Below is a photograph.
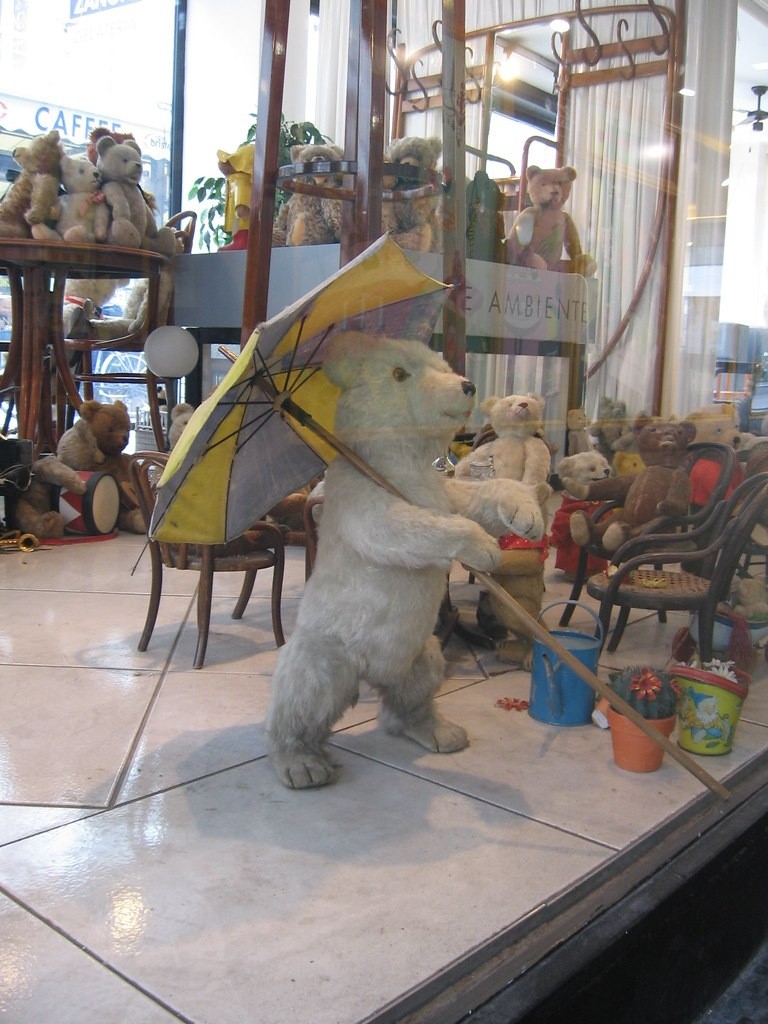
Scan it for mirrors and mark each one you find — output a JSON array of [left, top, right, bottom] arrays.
[[482, 17, 570, 181]]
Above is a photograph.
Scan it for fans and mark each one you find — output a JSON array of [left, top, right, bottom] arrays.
[[731, 86, 768, 133]]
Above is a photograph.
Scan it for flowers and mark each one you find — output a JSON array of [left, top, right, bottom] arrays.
[[606, 668, 679, 718], [679, 657, 739, 685]]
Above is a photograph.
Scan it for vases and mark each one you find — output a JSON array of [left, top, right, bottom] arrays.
[[671, 666, 752, 754], [605, 704, 677, 773]]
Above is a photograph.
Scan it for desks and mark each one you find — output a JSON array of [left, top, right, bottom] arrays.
[[0, 236, 167, 462]]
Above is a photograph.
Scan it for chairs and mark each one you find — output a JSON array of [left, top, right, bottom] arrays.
[[127, 449, 327, 671], [559, 441, 768, 666], [0, 211, 198, 452]]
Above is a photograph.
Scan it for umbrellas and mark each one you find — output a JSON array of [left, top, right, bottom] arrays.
[[147, 233, 735, 806]]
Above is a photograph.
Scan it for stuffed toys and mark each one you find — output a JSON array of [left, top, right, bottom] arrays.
[[270, 332, 543, 790], [0, 129, 767, 640]]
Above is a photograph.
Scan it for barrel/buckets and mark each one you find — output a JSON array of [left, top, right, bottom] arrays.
[[674, 661, 748, 756]]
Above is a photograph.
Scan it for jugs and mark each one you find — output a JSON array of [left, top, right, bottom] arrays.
[[525, 599, 605, 727]]
